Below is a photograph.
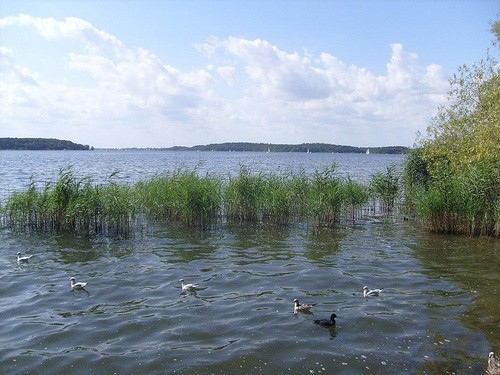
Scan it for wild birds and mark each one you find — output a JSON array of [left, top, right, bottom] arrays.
[[313, 313, 339, 327], [17, 251, 29, 263], [363, 285, 384, 298], [179, 279, 199, 295], [293, 298, 318, 313], [69, 276, 87, 291], [487, 351, 500, 375]]
